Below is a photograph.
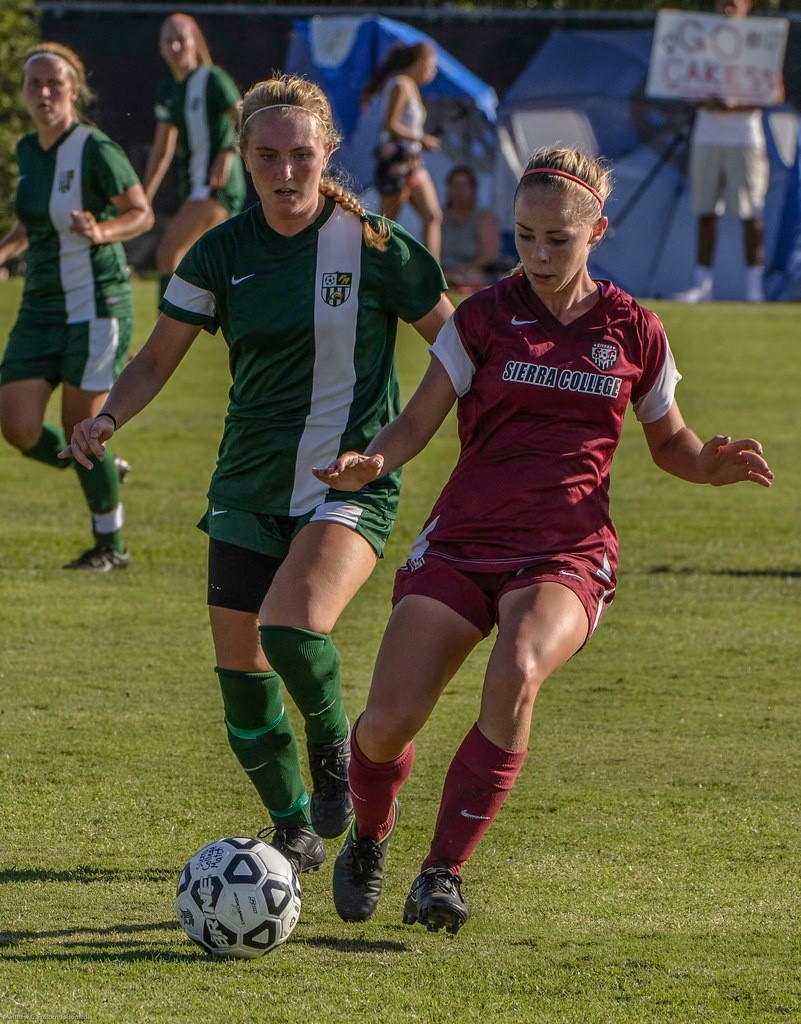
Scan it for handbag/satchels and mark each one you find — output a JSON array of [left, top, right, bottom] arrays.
[[372, 137, 421, 196]]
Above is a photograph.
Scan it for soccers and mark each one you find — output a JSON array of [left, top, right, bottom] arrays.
[[176, 837, 301, 961]]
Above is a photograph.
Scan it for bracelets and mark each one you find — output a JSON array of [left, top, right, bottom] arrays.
[[94, 412, 118, 431]]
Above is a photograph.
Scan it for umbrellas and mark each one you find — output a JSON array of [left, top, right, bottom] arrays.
[[283, 14, 527, 290], [495, 32, 800, 301]]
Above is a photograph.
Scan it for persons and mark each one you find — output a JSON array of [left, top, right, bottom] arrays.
[[359, 37, 444, 270], [57, 79, 458, 873], [141, 14, 246, 316], [357, 130, 500, 297], [311, 149, 775, 937], [672, 0, 786, 306], [0, 42, 155, 575]]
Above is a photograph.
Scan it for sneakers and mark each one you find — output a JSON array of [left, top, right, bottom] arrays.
[[745, 279, 765, 303], [673, 276, 712, 304], [333, 795, 399, 922], [309, 717, 354, 836], [270, 824, 326, 873], [113, 456, 130, 484], [401, 869, 470, 936], [61, 546, 129, 572]]
